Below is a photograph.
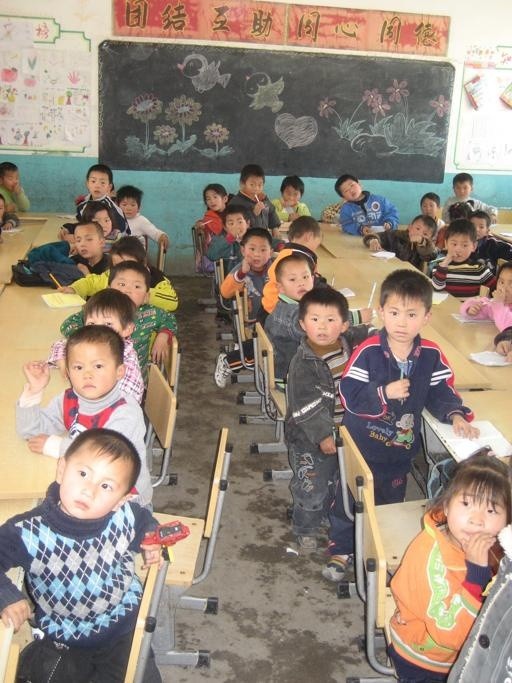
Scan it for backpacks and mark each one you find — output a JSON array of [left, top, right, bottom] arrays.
[[194, 219, 215, 273], [11, 258, 51, 288]]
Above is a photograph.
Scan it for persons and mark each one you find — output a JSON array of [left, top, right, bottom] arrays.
[[0, 426, 166, 683], [282, 287, 383, 553], [13, 325, 157, 513], [444, 519, 512, 682], [386, 444, 512, 683], [322, 267, 482, 582]]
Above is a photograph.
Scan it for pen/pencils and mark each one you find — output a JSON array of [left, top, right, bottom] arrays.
[[47, 271, 62, 288], [368, 281, 377, 308], [401, 368, 404, 405], [332, 272, 335, 286], [255, 194, 260, 202], [202, 220, 212, 225]]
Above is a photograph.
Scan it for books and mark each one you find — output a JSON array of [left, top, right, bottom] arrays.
[[434, 415, 510, 463]]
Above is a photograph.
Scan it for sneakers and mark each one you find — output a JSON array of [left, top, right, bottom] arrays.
[[214, 353, 232, 389], [297, 534, 317, 555], [214, 314, 235, 332], [322, 552, 354, 582]]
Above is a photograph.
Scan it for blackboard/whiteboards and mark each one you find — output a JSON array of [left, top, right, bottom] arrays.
[[99, 41, 456, 185]]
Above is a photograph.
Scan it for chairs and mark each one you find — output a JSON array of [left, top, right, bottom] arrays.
[[125, 427, 233, 683], [156, 239, 168, 273], [192, 221, 225, 314], [214, 257, 257, 384], [137, 335, 181, 488], [333, 425, 435, 678], [236, 321, 295, 481]]
